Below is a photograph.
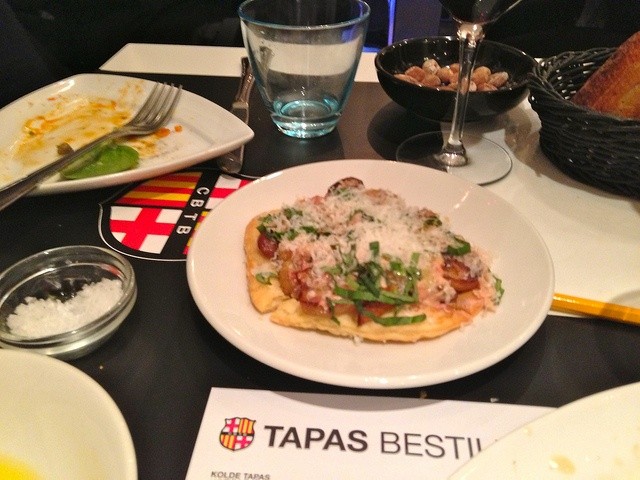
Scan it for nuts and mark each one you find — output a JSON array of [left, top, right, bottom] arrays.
[[394, 57, 510, 94]]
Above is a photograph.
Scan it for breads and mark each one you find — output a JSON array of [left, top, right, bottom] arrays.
[[570, 30, 639, 121]]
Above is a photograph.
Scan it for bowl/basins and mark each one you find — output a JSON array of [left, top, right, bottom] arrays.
[[374, 35, 538, 124], [0, 244, 136, 360]]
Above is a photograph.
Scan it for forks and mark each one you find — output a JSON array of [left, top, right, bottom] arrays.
[[0, 82, 183, 210]]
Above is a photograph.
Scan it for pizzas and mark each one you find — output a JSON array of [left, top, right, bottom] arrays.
[[243, 177, 506, 344]]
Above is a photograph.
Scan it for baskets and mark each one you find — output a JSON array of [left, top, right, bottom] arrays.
[[526, 46, 639, 200]]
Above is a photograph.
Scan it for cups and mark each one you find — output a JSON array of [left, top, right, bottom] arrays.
[[395, 0, 519, 187], [238, 1, 372, 140]]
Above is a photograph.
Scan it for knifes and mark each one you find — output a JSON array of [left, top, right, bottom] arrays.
[[220, 65, 251, 176]]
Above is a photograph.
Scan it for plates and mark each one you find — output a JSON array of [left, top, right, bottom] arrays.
[[447, 381, 640, 480], [0, 349, 138, 480], [2, 73, 257, 197], [185, 158, 555, 390]]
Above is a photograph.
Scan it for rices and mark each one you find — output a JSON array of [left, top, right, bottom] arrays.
[[6, 275, 124, 339]]
